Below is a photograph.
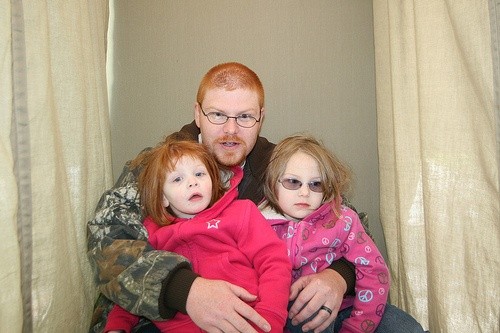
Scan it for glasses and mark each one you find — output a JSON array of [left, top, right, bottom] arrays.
[[198, 101, 262, 128], [277, 179, 326, 193]]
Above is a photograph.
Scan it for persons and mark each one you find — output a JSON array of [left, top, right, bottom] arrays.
[[100, 139, 291, 333], [82, 62, 427, 333], [254, 134, 393, 333]]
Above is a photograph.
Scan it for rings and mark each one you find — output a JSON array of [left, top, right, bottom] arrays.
[[320, 306, 333, 314]]
